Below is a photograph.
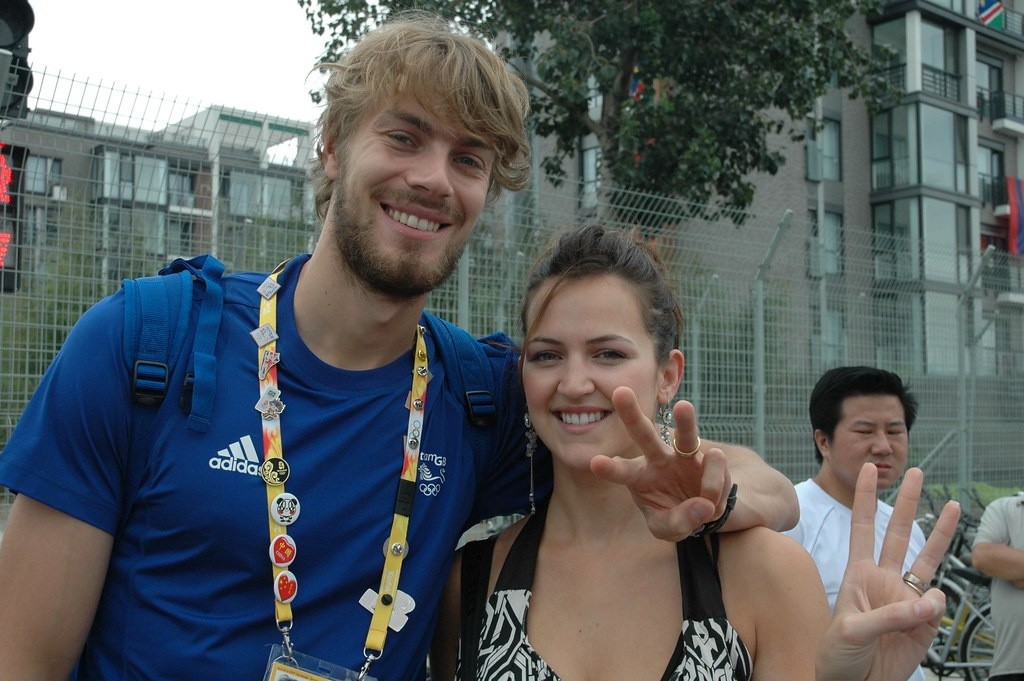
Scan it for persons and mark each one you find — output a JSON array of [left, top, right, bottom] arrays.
[[779, 366, 926, 681], [0, 22, 800, 681], [427, 223, 961, 681], [972, 492, 1024, 681]]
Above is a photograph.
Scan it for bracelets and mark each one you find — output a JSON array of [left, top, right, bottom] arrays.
[[690, 483, 738, 536]]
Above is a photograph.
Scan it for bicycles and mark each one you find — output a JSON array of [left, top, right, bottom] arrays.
[[894, 482, 1024, 681]]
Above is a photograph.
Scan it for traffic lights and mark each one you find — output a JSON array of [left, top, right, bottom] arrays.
[[1, 0, 35, 119], [0, 139, 31, 294]]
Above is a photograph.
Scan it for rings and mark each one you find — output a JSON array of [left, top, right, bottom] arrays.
[[903, 571, 931, 595], [672, 437, 701, 456]]
